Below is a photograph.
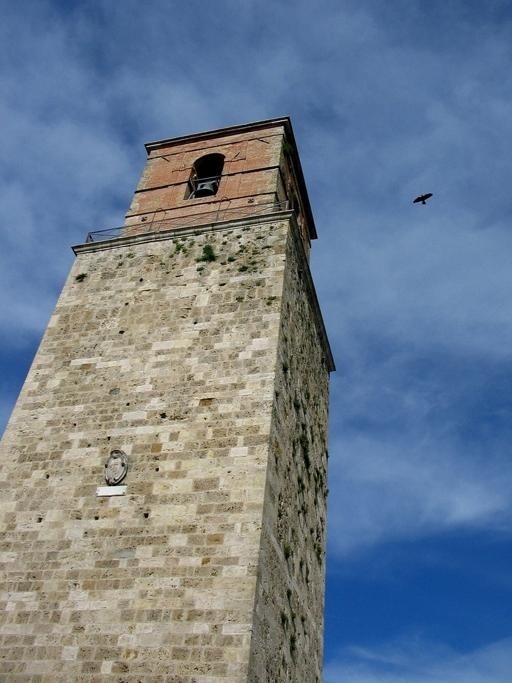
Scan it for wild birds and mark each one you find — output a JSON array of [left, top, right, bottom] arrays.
[[413, 193, 432, 204]]
